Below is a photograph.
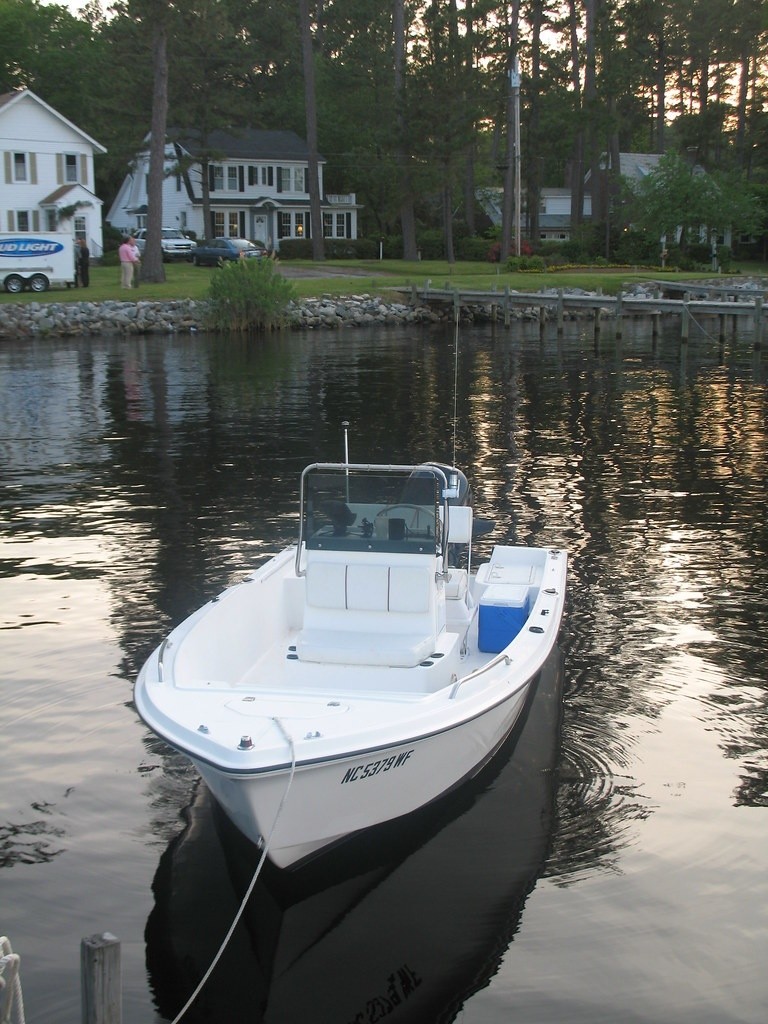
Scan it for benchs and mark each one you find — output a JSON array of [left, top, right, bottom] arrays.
[[297, 563, 437, 668], [440, 506, 472, 616], [475, 563, 542, 602]]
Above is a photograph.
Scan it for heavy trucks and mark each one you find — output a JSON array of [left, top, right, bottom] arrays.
[[0, 228, 74, 293]]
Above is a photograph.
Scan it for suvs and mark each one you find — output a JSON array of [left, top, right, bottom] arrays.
[[132, 225, 198, 262]]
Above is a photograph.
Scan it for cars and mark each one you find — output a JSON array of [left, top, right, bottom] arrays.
[[189, 235, 271, 270]]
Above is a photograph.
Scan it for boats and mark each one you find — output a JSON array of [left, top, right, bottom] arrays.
[[144, 641, 564, 1024], [132, 421, 571, 874]]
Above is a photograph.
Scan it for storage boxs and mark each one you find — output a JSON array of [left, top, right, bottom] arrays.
[[478, 585, 531, 654]]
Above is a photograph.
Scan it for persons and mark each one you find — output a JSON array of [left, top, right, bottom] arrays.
[[66, 238, 89, 288], [119, 235, 141, 290]]
